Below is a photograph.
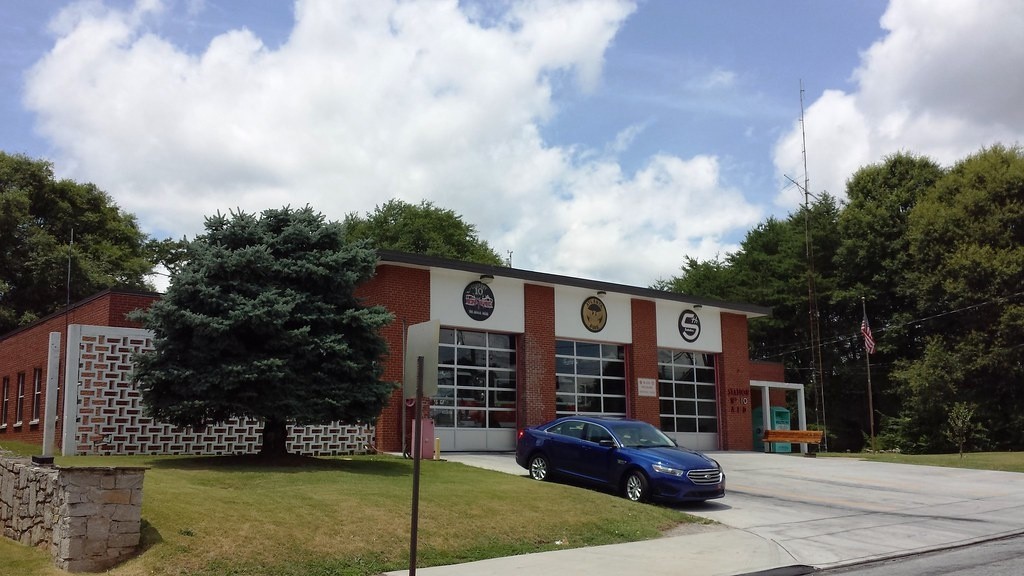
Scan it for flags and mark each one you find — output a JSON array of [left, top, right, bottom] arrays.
[[860, 314, 875, 355]]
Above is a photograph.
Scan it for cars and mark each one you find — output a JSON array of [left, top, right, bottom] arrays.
[[516, 416, 726, 505]]
